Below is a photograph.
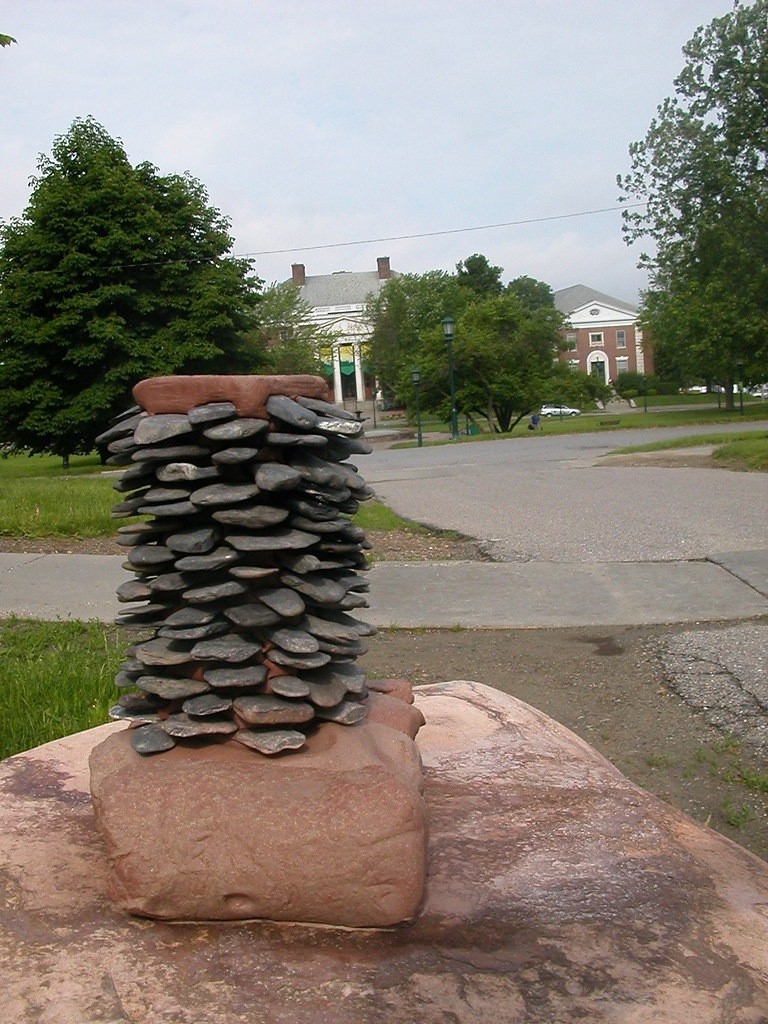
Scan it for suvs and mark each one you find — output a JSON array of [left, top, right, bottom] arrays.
[[539, 404, 581, 420]]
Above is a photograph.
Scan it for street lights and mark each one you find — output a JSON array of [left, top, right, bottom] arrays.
[[642, 375, 648, 412], [736, 358, 743, 412], [440, 317, 461, 441], [372, 387, 379, 430], [411, 367, 423, 448]]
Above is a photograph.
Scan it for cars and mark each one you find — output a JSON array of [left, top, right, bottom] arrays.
[[686, 382, 768, 399]]
